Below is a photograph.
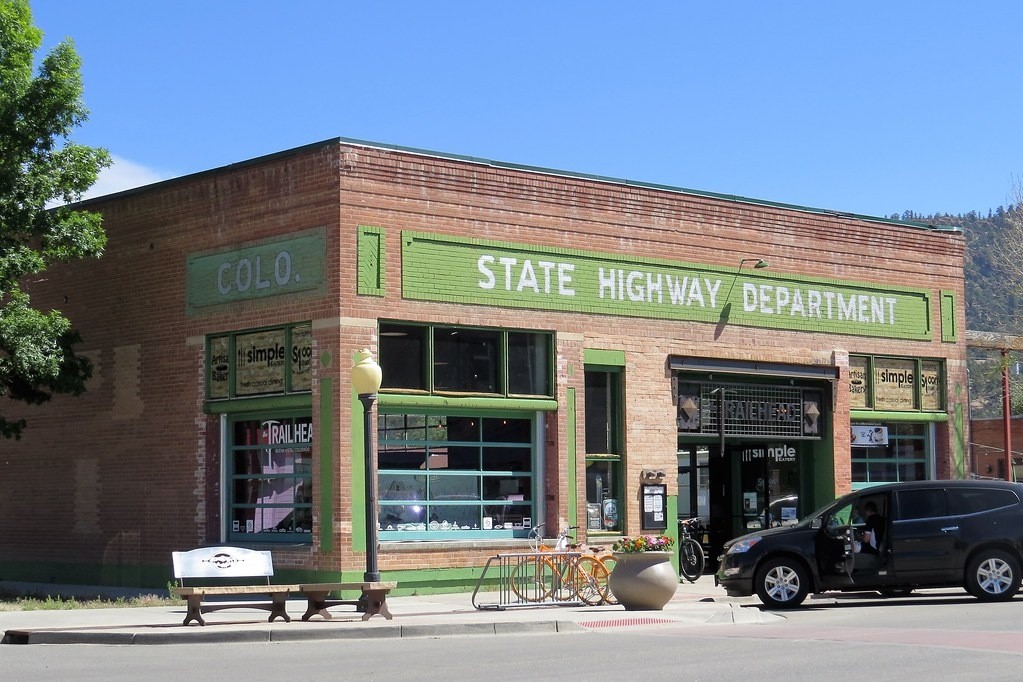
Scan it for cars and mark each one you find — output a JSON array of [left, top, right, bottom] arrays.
[[748, 493, 799, 529]]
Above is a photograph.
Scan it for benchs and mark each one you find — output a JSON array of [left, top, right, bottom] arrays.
[[302, 581, 397, 621], [171, 546, 300, 625]]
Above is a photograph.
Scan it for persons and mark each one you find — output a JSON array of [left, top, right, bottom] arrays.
[[853, 499, 884, 555]]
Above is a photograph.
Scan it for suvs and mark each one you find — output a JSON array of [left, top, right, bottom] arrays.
[[714, 479, 1023, 610]]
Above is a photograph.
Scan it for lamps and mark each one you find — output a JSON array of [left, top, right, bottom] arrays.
[[743, 259, 771, 269]]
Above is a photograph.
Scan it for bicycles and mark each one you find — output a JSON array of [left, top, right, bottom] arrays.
[[509, 520, 622, 606], [676, 515, 705, 581]]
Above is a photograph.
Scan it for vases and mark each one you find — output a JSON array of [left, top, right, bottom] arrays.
[[609, 551, 677, 611]]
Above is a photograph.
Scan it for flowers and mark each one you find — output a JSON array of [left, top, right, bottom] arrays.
[[613, 534, 674, 553]]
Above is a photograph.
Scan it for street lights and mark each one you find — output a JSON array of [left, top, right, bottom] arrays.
[[350, 346, 388, 613]]
